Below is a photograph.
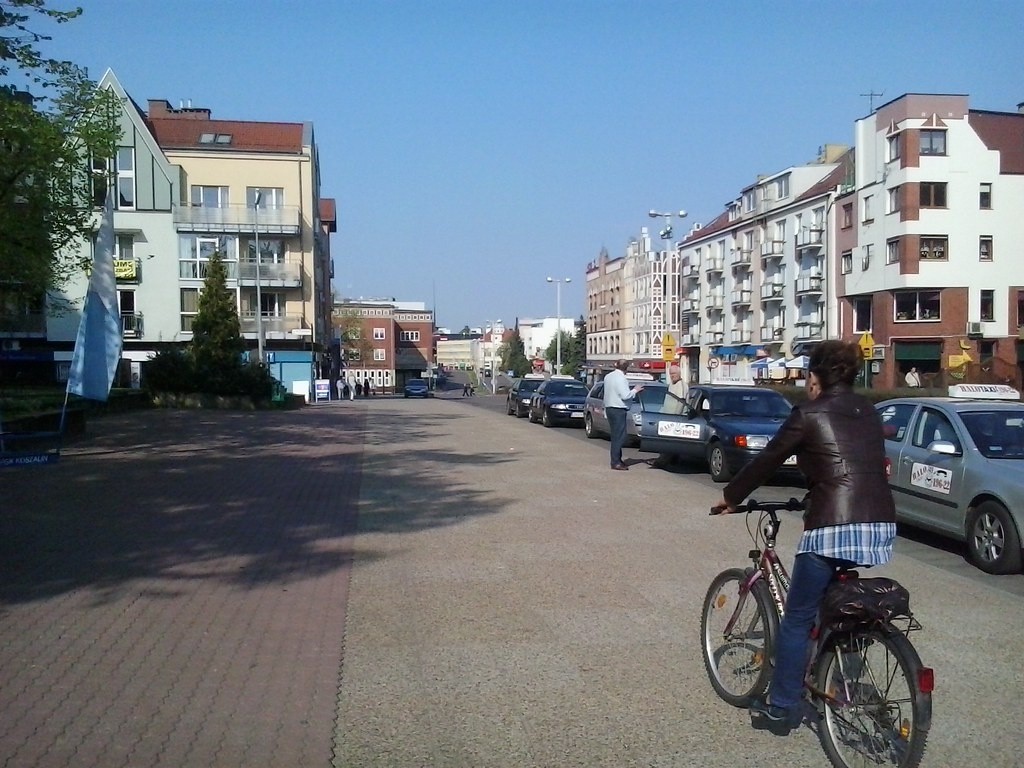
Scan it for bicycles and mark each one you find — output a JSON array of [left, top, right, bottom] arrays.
[[700, 496, 934, 768]]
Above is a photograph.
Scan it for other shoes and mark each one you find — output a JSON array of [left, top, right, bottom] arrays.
[[647, 458, 657, 464], [611, 461, 629, 470]]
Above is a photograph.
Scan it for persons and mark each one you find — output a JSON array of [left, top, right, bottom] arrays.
[[905, 368, 921, 388], [337, 373, 375, 401], [719, 340, 897, 725], [462, 383, 477, 397], [651, 365, 689, 466], [604, 358, 644, 470]]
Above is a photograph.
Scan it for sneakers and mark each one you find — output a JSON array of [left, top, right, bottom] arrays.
[[748, 693, 790, 721]]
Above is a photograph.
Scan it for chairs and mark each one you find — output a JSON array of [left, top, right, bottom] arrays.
[[746, 401, 758, 411], [934, 421, 957, 444], [702, 397, 724, 410]]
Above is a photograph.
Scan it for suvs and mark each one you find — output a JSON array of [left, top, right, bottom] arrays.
[[585, 380, 669, 448]]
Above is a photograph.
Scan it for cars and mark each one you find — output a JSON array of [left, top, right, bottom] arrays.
[[529, 379, 590, 428], [637, 384, 808, 484], [404, 379, 429, 398], [871, 396, 1024, 576], [506, 378, 546, 418], [480, 368, 491, 377]]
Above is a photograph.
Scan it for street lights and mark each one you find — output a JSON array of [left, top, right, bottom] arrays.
[[546, 277, 572, 375], [648, 208, 690, 385], [254, 189, 264, 367], [487, 319, 502, 393]]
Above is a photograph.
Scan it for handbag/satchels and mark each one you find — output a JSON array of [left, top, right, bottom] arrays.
[[819, 576, 910, 631]]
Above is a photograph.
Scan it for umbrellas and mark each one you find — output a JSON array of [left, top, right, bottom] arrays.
[[751, 354, 811, 378]]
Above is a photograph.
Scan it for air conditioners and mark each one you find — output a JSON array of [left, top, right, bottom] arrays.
[[966, 321, 985, 335]]
